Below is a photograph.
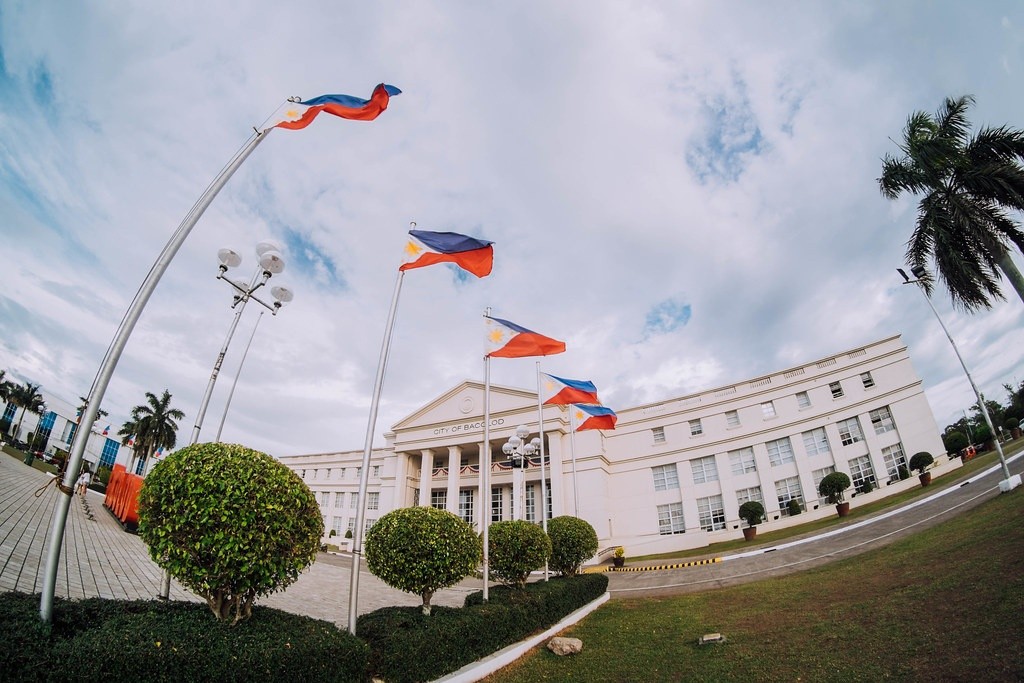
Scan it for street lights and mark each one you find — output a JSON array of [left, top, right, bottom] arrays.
[[893, 262, 1021, 494], [156, 237, 294, 600], [502, 425, 542, 520]]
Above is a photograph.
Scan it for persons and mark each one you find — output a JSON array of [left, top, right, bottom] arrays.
[[75, 461, 99, 496]]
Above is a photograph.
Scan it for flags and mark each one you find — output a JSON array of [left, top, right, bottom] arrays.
[[399, 230, 496, 278], [484, 315, 566, 357], [102, 425, 110, 435], [128, 434, 136, 445], [154, 445, 164, 457], [540, 372, 598, 405], [573, 404, 617, 434], [265, 83, 402, 129], [77, 411, 84, 422]]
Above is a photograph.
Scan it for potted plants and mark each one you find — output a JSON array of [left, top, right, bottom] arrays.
[[819, 471, 850, 518], [612, 547, 626, 568], [1005, 417, 1020, 441], [908, 452, 934, 487], [738, 501, 765, 541]]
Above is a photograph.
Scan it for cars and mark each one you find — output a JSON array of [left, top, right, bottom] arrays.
[[699, 632, 726, 646], [1, 431, 101, 483]]
